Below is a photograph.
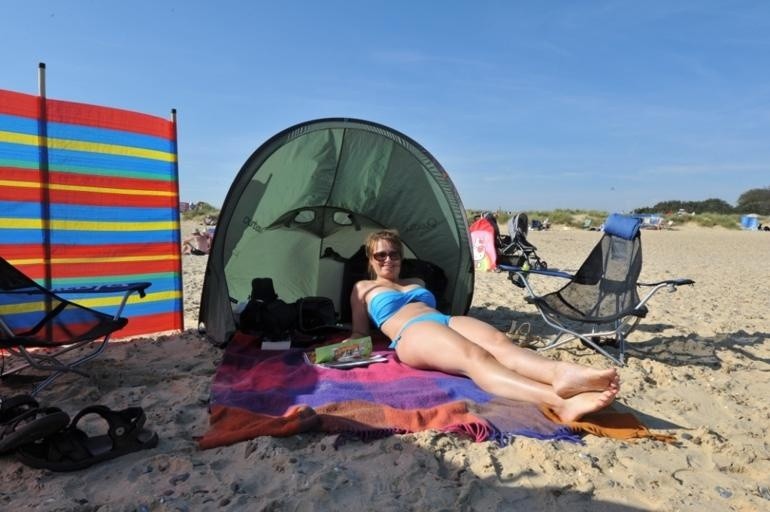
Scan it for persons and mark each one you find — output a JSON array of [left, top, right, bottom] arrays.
[[308, 228, 621, 430], [180, 227, 214, 258]]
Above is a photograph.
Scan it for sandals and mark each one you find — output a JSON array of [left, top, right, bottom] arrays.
[[0, 392, 72, 457], [16, 402, 161, 474], [505, 322, 552, 350]]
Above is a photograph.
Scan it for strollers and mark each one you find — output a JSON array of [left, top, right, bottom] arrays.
[[473, 212, 547, 270]]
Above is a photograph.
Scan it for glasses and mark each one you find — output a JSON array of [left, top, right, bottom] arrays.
[[371, 251, 403, 263]]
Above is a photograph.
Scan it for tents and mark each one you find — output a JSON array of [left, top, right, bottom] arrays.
[[194, 113, 475, 348]]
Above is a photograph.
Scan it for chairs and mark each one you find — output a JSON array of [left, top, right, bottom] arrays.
[[0, 255, 151, 398], [497, 213, 697, 367]]
